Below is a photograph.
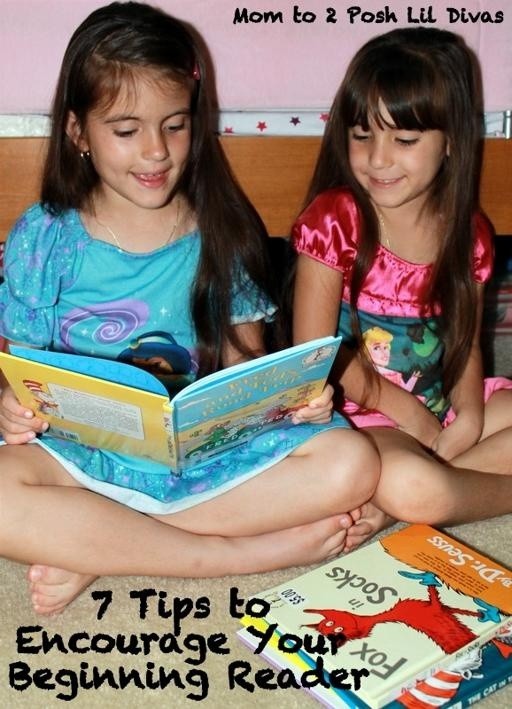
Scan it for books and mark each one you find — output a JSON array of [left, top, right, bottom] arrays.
[[231, 525, 512, 708], [1, 334, 344, 476]]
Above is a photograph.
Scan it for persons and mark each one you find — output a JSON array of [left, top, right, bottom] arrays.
[[0, 1, 383, 615], [361, 326, 423, 392], [283, 26, 511, 531]]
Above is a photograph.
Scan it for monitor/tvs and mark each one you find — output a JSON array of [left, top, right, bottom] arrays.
[[1, 334, 344, 476]]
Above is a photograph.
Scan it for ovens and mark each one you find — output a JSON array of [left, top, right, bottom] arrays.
[[0, 136, 510, 707]]
